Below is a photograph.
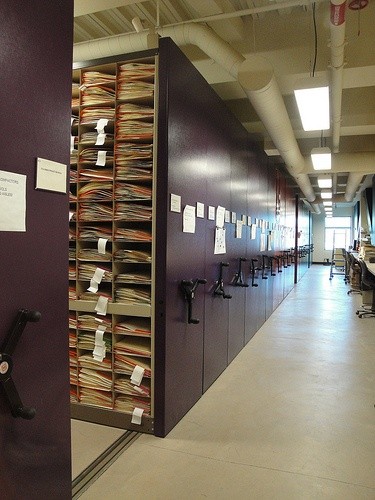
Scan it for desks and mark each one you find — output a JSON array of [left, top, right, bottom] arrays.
[[350, 253, 375, 275]]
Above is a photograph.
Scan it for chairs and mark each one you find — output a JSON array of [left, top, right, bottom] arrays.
[[330, 248, 347, 280], [356, 261, 375, 318], [347, 255, 362, 295]]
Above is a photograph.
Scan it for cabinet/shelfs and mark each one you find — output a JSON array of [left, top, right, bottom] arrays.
[[0, 0, 311, 500]]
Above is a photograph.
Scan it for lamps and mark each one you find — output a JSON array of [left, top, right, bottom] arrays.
[[294, 2, 332, 132], [311, 130, 332, 171], [318, 173, 332, 218]]
